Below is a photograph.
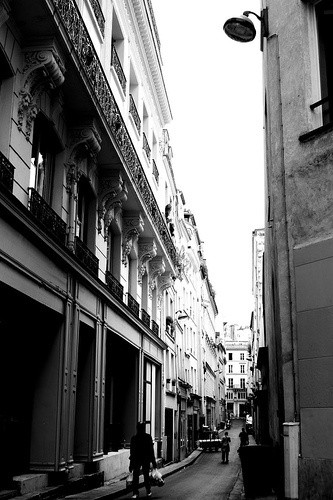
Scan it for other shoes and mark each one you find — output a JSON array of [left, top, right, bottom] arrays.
[[226, 460, 229, 464], [131, 493, 140, 499], [147, 491, 153, 497], [221, 459, 225, 463]]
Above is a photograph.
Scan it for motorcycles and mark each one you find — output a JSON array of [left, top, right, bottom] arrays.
[[226, 419, 232, 429]]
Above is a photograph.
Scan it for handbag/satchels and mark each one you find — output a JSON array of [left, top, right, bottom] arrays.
[[149, 468, 165, 487]]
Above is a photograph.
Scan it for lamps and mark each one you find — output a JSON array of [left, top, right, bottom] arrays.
[[223, 6, 270, 43], [175, 310, 188, 321], [246, 354, 255, 359]]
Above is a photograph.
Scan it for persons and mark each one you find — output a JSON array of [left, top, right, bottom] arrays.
[[221, 432, 231, 464], [239, 427, 249, 447], [129, 421, 156, 498]]
